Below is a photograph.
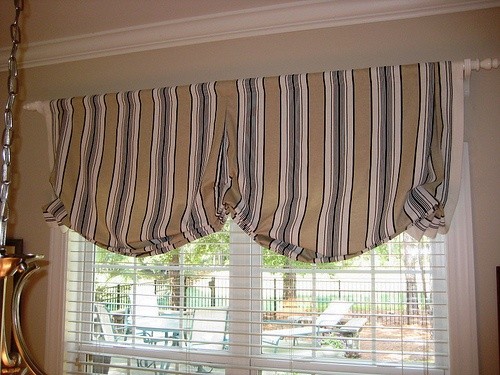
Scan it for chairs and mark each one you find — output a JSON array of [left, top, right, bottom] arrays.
[[129, 292, 160, 320], [94, 303, 126, 342], [186, 308, 227, 350], [261, 299, 354, 355]]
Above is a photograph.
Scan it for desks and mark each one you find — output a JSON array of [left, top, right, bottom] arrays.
[[129, 317, 191, 371]]
